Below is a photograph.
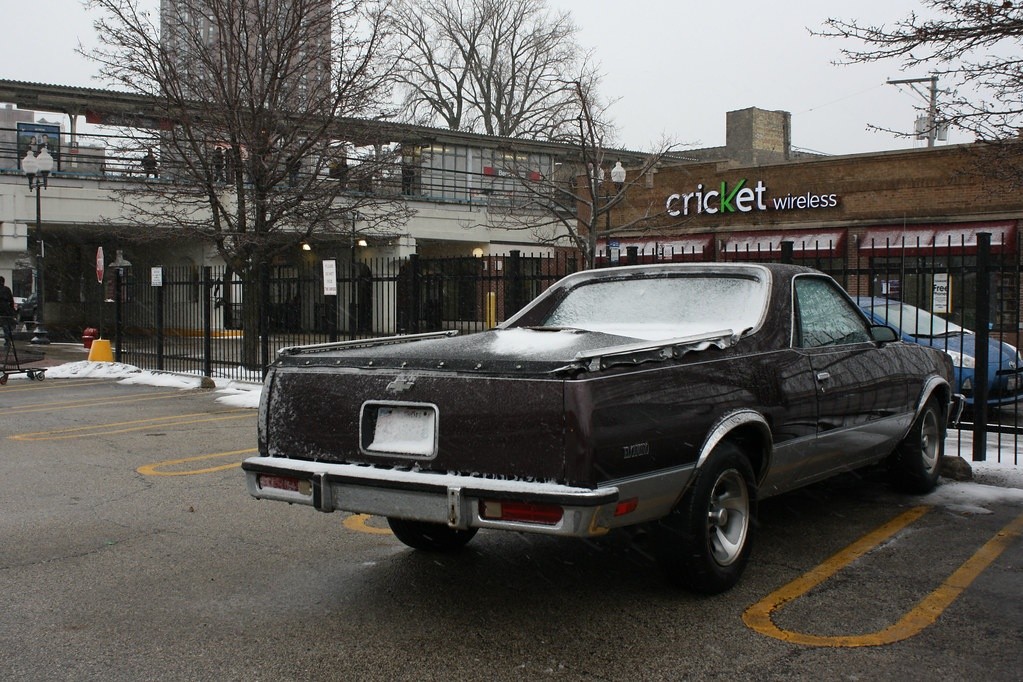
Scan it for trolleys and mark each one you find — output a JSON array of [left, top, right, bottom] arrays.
[[0, 313, 48, 386]]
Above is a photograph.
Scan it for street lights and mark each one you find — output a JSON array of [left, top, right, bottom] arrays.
[[590, 161, 627, 267], [21, 148, 55, 345]]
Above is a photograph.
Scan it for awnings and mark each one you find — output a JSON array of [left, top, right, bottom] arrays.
[[721, 228, 847, 258], [595, 233, 714, 261], [859, 220, 1016, 257]]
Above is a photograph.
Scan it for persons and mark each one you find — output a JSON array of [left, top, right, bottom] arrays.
[[402, 163, 414, 195], [39, 134, 54, 156], [0, 276, 16, 345], [287, 152, 299, 186], [141, 148, 158, 178], [329, 157, 349, 189], [212, 147, 226, 182], [27, 136, 39, 156]]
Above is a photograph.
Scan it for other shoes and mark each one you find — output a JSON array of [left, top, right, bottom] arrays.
[[4, 343, 13, 348]]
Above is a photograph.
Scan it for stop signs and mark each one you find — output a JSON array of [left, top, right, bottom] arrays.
[[95, 246, 105, 284]]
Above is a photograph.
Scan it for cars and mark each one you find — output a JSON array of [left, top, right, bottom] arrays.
[[849, 296, 1023, 407], [242, 260, 967, 596], [16, 294, 38, 327]]
